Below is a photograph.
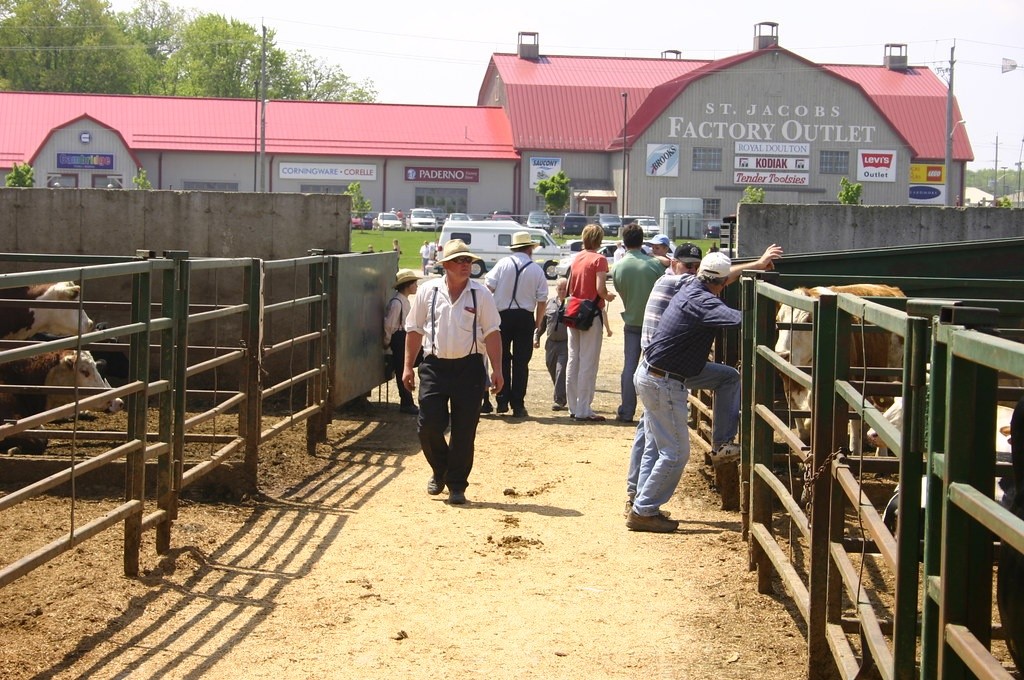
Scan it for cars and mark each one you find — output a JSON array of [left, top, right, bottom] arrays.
[[629, 219, 661, 237], [443, 213, 473, 221], [482, 210, 514, 220], [555, 239, 677, 281], [405, 208, 437, 232], [350, 210, 365, 229], [378, 213, 402, 231], [362, 213, 372, 230], [562, 212, 587, 234], [705, 221, 721, 238]]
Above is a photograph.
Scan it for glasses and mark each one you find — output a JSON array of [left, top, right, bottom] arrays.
[[681, 262, 699, 270], [452, 256, 473, 263]]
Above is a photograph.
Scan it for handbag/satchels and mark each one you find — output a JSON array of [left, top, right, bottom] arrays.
[[554, 296, 600, 331]]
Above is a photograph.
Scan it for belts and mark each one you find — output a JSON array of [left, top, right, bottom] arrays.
[[641, 357, 685, 383]]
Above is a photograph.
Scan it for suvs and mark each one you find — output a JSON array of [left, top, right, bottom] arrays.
[[593, 212, 620, 237]]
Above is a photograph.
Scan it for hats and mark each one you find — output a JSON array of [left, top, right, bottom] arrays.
[[673, 243, 702, 263], [436, 239, 480, 264], [509, 232, 538, 250], [648, 234, 670, 247], [393, 270, 423, 288], [699, 251, 731, 277]]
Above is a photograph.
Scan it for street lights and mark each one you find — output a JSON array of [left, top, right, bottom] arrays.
[[260, 98, 272, 192], [1001, 166, 1008, 195], [1015, 162, 1024, 207], [620, 92, 628, 237]]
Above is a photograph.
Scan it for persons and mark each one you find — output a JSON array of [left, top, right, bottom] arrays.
[[368, 244, 374, 252], [485, 232, 548, 418], [371, 218, 380, 231], [955, 194, 978, 207], [402, 238, 503, 503], [390, 208, 411, 231], [384, 269, 419, 413], [612, 224, 782, 533], [533, 277, 568, 410], [393, 239, 402, 272], [567, 223, 616, 424]]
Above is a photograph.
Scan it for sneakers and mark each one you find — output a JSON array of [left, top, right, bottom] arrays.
[[711, 441, 740, 463], [624, 501, 679, 532]]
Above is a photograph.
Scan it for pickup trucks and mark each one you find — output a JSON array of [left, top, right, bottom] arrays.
[[527, 211, 553, 234]]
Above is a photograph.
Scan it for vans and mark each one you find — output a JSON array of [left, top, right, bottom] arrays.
[[425, 220, 560, 280]]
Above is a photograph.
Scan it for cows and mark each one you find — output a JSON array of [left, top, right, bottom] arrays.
[[866, 381, 1015, 509], [775, 283, 907, 475], [0, 280, 126, 456]]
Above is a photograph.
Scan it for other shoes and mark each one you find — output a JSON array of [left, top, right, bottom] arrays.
[[427, 469, 446, 495], [401, 404, 419, 414], [570, 413, 605, 422], [481, 402, 493, 413], [448, 488, 466, 504], [552, 402, 567, 410], [513, 408, 529, 417], [496, 396, 508, 413]]
